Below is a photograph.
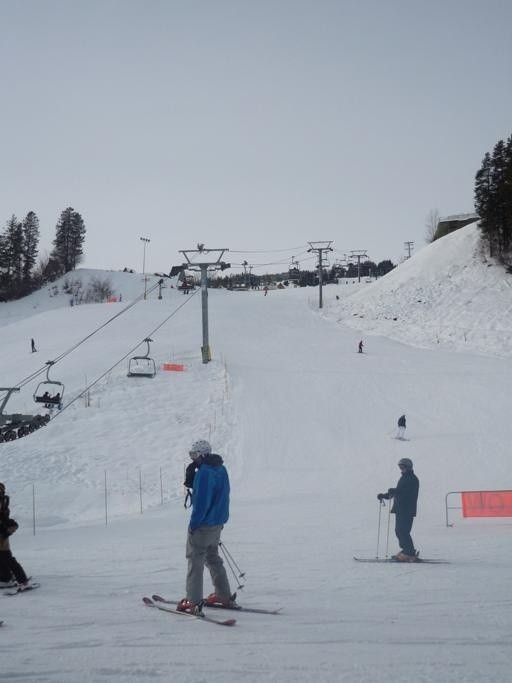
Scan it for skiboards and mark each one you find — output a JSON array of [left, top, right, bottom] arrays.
[[0, 575, 36, 596], [353, 555, 451, 565], [143, 596, 282, 626]]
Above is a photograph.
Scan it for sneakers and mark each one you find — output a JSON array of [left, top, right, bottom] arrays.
[[178, 599, 196, 611], [208, 593, 229, 605], [0, 580, 30, 592], [393, 552, 416, 561]]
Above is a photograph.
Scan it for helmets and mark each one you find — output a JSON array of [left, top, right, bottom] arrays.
[[399, 459, 412, 471], [189, 441, 212, 465]]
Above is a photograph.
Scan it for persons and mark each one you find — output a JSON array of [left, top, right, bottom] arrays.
[[262, 284, 270, 297], [30, 337, 38, 354], [0, 485, 29, 591], [42, 391, 50, 409], [175, 438, 230, 609], [357, 340, 366, 353], [52, 392, 62, 410], [394, 412, 408, 438], [376, 456, 420, 560]]
